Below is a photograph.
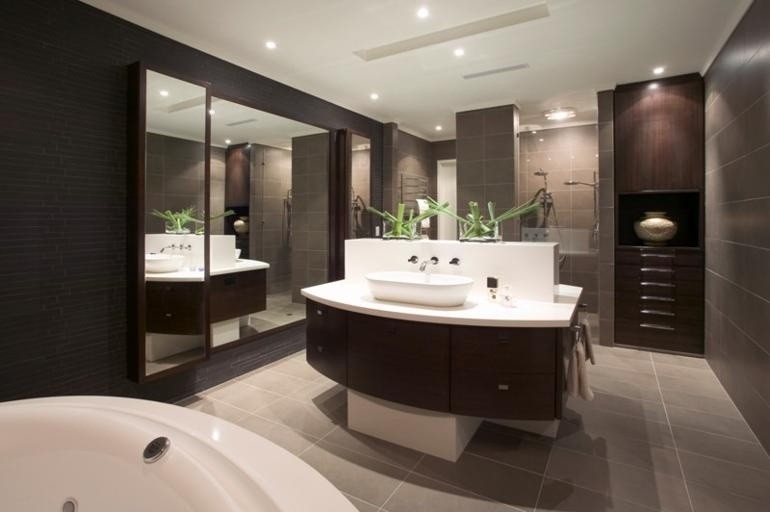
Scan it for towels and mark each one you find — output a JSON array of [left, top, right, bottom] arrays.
[[566, 318, 597, 403]]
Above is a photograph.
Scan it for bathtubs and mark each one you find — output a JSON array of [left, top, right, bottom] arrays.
[[1, 393, 357, 512]]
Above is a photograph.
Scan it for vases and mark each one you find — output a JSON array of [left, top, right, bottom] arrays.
[[632, 209, 679, 243]]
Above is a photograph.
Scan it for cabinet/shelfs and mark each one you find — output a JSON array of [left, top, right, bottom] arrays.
[[613, 73, 706, 357], [146, 269, 266, 335], [305, 297, 577, 421]]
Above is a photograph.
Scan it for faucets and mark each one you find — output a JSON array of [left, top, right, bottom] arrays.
[[159, 244, 182, 252], [418, 258, 439, 272]]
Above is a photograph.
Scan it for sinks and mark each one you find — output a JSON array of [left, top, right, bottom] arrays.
[[365, 269, 476, 306], [144, 252, 185, 273]]
